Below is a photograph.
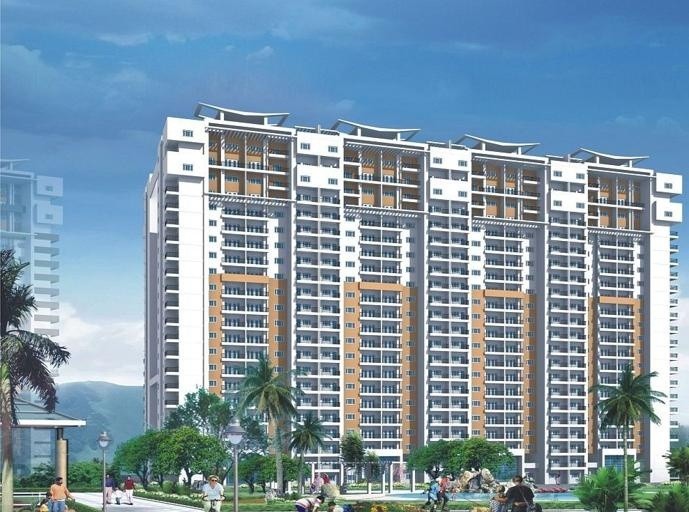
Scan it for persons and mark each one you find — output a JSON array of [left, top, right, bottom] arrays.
[[487, 485, 530, 511], [50, 477, 74, 511], [327, 501, 344, 512], [105, 474, 116, 504], [123, 474, 136, 505], [420, 477, 443, 511], [434, 474, 454, 512], [295, 495, 324, 512], [46, 491, 53, 512], [492, 474, 534, 512], [39, 498, 49, 512], [310, 473, 330, 495], [201, 474, 225, 511], [114, 486, 123, 505]]
